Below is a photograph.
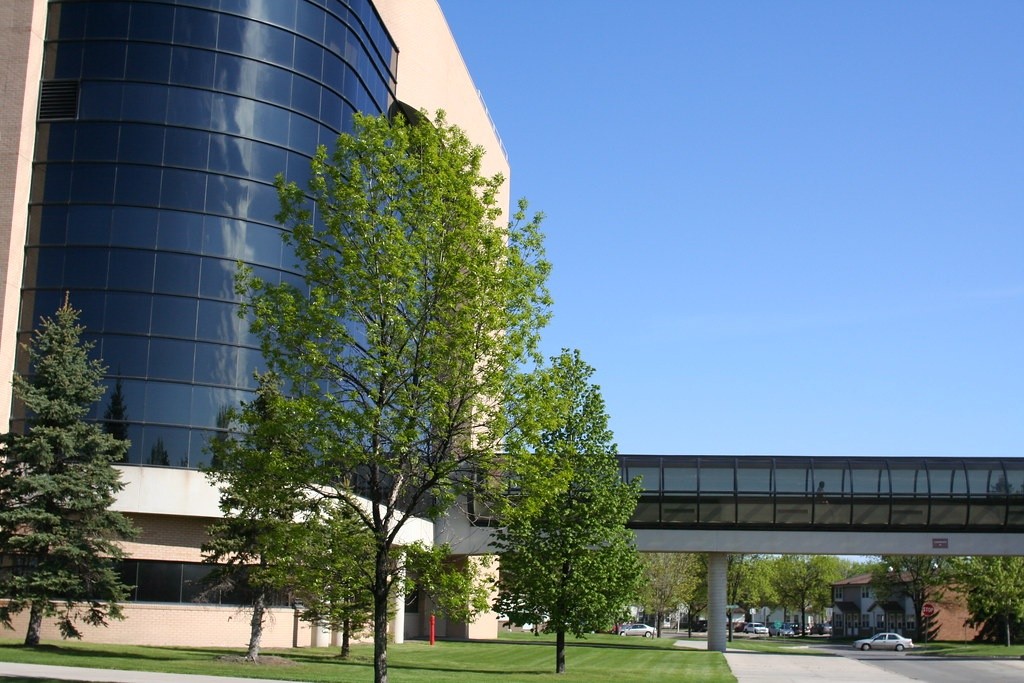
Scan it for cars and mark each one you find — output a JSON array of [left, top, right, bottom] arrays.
[[789, 622, 811, 635], [744, 623, 768, 634], [810, 623, 833, 635], [610, 623, 622, 635], [690, 620, 708, 632], [734, 621, 747, 632], [619, 623, 658, 638], [853, 632, 914, 651], [766, 622, 794, 637]]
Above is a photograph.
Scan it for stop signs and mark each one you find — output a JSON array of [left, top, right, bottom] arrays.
[[921, 602, 934, 617]]
